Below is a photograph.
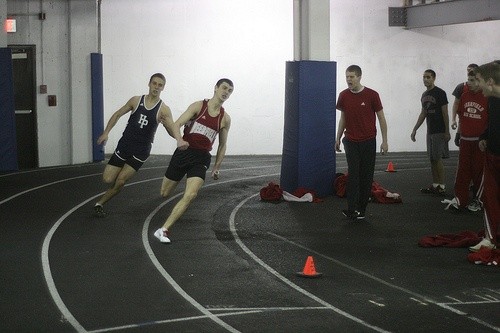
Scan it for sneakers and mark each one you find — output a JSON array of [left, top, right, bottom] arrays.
[[464, 246, 500, 264], [421, 185, 445, 193], [469, 239, 496, 251], [153, 228, 171, 243], [465, 202, 481, 211], [93, 204, 104, 214], [341, 209, 365, 219]]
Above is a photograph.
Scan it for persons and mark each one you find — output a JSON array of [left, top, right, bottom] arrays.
[[410, 69, 451, 195], [334, 65, 388, 220], [92, 73, 176, 217], [153, 78, 234, 243], [441, 60, 500, 266]]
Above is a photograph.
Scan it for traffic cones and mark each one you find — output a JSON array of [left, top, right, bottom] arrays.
[[384, 160, 397, 172], [297, 256, 323, 278]]
